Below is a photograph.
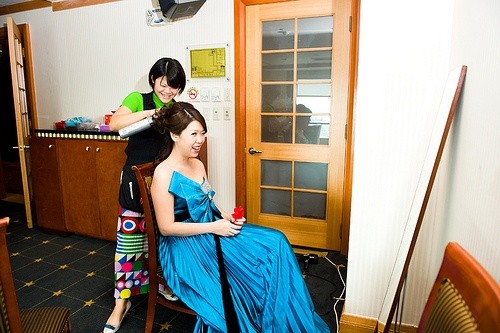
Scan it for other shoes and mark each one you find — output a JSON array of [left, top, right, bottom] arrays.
[[104, 300, 131, 333], [159, 285, 179, 300]]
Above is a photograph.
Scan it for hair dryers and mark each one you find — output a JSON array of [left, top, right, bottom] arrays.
[[297, 253, 319, 273]]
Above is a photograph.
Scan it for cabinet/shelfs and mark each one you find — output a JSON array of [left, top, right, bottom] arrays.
[[28, 138, 208, 241]]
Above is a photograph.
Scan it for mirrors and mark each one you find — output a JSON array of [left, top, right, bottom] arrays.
[[373, 65, 468, 333]]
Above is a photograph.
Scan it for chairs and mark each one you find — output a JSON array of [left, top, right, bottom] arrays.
[[416, 242, 500, 333], [131, 155, 197, 333], [0, 216, 72, 333]]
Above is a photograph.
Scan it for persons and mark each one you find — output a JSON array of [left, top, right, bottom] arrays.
[[285, 104, 328, 192], [104, 57, 186, 333], [262, 84, 291, 187], [150, 101, 331, 333]]
